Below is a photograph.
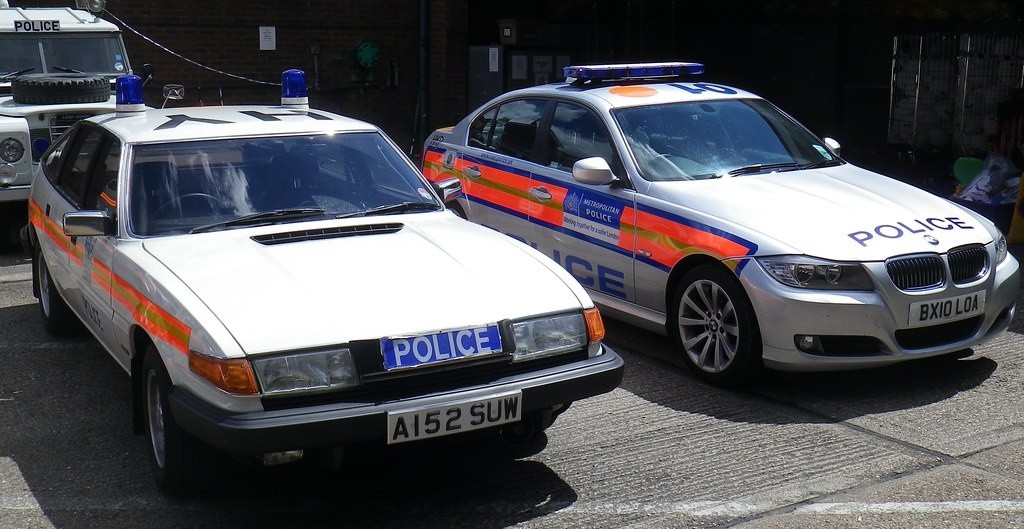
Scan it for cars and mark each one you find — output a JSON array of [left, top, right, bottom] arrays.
[[421, 63, 1023, 391], [15, 69, 627, 499]]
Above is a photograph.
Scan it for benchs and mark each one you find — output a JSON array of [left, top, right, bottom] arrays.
[[118, 161, 325, 217], [489, 121, 658, 156]]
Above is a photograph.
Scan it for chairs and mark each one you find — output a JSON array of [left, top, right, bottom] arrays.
[[261, 152, 328, 203], [132, 161, 197, 219], [563, 113, 604, 166]]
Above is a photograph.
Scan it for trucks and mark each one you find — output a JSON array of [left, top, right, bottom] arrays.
[[0, 0, 152, 206]]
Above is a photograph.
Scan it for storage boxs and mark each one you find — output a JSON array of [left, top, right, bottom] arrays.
[[959, 198, 1015, 235]]
